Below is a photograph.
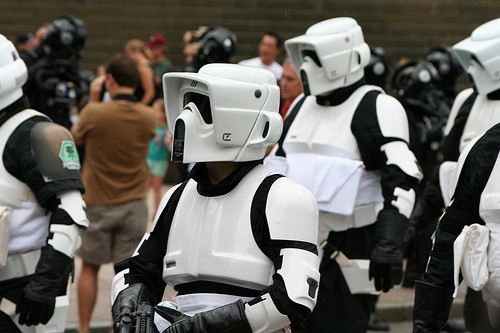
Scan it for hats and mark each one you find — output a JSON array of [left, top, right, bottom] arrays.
[[147, 34, 166, 49]]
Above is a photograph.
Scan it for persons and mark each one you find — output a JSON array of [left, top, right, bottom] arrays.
[[19, 17, 91, 133], [0, 33, 88, 333], [111, 63, 320, 333], [268, 16, 500, 333], [71, 30, 203, 333], [238, 32, 303, 123], [191, 26, 238, 69]]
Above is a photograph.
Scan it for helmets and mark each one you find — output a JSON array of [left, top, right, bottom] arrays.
[[366, 44, 462, 117], [0, 32, 29, 110], [283, 16, 371, 97], [161, 63, 285, 164], [191, 26, 241, 69], [31, 14, 88, 63], [452, 18, 500, 95]]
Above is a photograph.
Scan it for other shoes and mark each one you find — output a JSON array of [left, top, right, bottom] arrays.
[[367, 314, 391, 333]]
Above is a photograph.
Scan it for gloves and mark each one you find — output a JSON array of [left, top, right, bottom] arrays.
[[412, 279, 454, 333], [151, 297, 254, 333], [402, 183, 445, 259], [15, 246, 74, 327], [368, 209, 410, 293], [111, 282, 149, 333]]
